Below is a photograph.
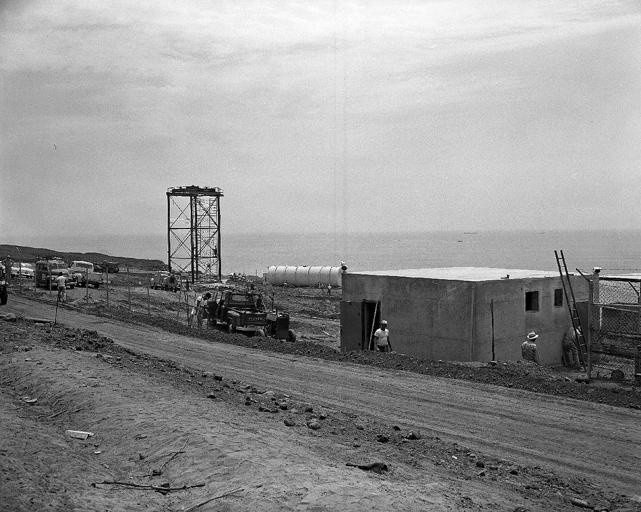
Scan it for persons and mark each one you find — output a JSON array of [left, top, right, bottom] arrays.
[[521, 332, 539, 364], [55, 272, 67, 302], [251, 284, 254, 290], [212, 246, 217, 257], [374, 320, 393, 352], [563, 326, 583, 369], [188, 292, 211, 326], [3, 255, 14, 282]]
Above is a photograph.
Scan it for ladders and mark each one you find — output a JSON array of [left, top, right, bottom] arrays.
[[554, 250, 588, 372]]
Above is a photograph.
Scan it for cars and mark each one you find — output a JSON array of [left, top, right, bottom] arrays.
[[149, 271, 177, 292], [0, 261, 35, 305]]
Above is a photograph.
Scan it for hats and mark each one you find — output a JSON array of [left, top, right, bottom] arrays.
[[527, 332, 539, 339], [379, 320, 387, 324]]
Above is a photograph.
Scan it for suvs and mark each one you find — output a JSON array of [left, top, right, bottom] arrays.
[[35, 257, 78, 290]]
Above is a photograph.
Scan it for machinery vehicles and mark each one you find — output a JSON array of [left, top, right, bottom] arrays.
[[207, 285, 297, 342]]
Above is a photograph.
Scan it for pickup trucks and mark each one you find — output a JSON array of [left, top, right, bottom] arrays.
[[68, 261, 102, 289]]
